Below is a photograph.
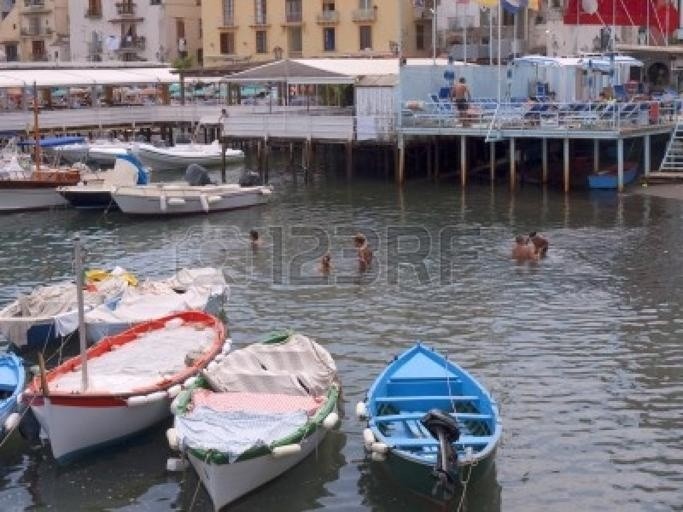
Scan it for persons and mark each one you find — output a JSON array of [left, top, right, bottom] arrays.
[[599, 92, 611, 102], [316, 254, 335, 275], [353, 234, 374, 277], [544, 92, 560, 121], [450, 75, 473, 127], [525, 230, 550, 259], [524, 94, 539, 108], [512, 234, 538, 261], [248, 230, 265, 245], [219, 107, 230, 120]]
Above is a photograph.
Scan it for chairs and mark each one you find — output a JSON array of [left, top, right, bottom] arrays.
[[427, 92, 675, 135]]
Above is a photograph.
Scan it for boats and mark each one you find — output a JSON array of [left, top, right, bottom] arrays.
[[174, 330, 344, 508], [588, 157, 641, 189], [0, 134, 274, 216], [361, 339, 504, 500], [1, 264, 230, 467]]
